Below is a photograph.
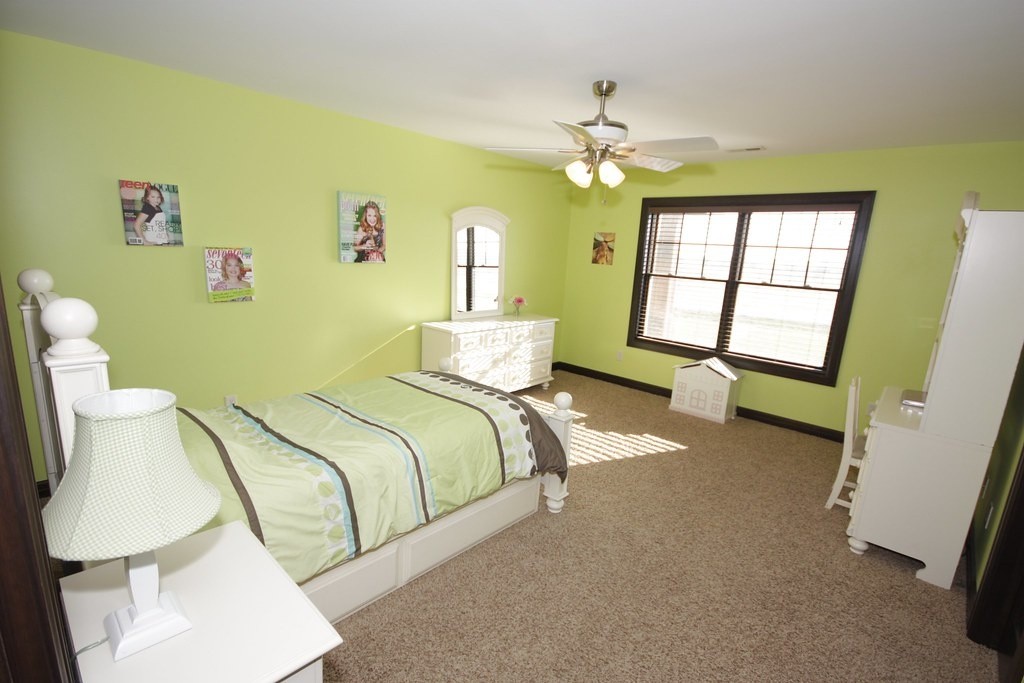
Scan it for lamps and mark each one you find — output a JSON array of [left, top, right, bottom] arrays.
[[565, 150, 625, 187], [40, 389, 206, 660]]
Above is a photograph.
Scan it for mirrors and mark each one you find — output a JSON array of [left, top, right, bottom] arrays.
[[450, 206, 511, 321]]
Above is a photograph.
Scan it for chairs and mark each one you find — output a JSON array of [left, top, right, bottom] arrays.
[[825, 374, 867, 511]]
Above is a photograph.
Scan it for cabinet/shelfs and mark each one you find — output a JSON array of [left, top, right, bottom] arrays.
[[419, 313, 560, 393], [846, 189, 1024, 591]]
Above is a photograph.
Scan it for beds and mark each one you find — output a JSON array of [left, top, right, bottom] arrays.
[[16, 267, 573, 625]]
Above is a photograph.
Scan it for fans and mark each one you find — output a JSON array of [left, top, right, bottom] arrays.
[[479, 80, 719, 173]]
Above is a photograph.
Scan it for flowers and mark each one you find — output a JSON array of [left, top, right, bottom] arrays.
[[508, 295, 527, 314]]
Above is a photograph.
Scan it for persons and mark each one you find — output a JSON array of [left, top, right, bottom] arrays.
[[215, 253, 253, 301], [354, 200, 387, 263], [133, 185, 169, 245]]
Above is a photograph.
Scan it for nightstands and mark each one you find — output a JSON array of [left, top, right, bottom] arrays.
[[59, 520, 344, 683]]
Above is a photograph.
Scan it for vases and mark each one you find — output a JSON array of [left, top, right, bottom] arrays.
[[512, 304, 520, 316]]
